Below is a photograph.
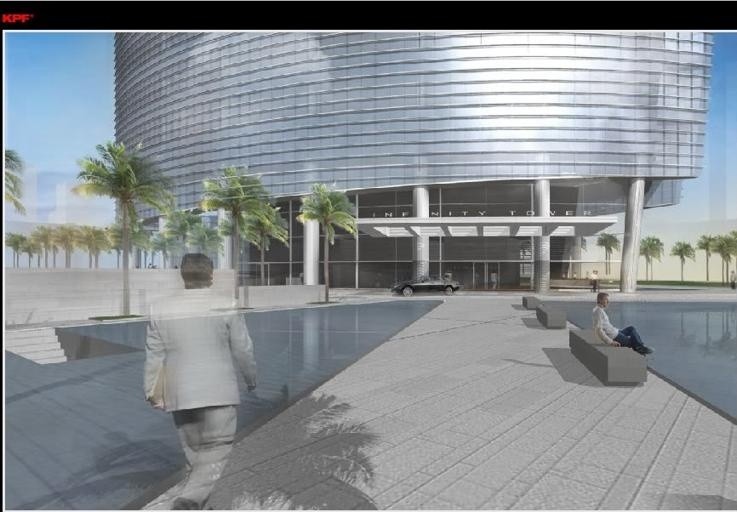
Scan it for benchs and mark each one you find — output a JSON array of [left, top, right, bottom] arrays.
[[521, 295, 647, 385]]
